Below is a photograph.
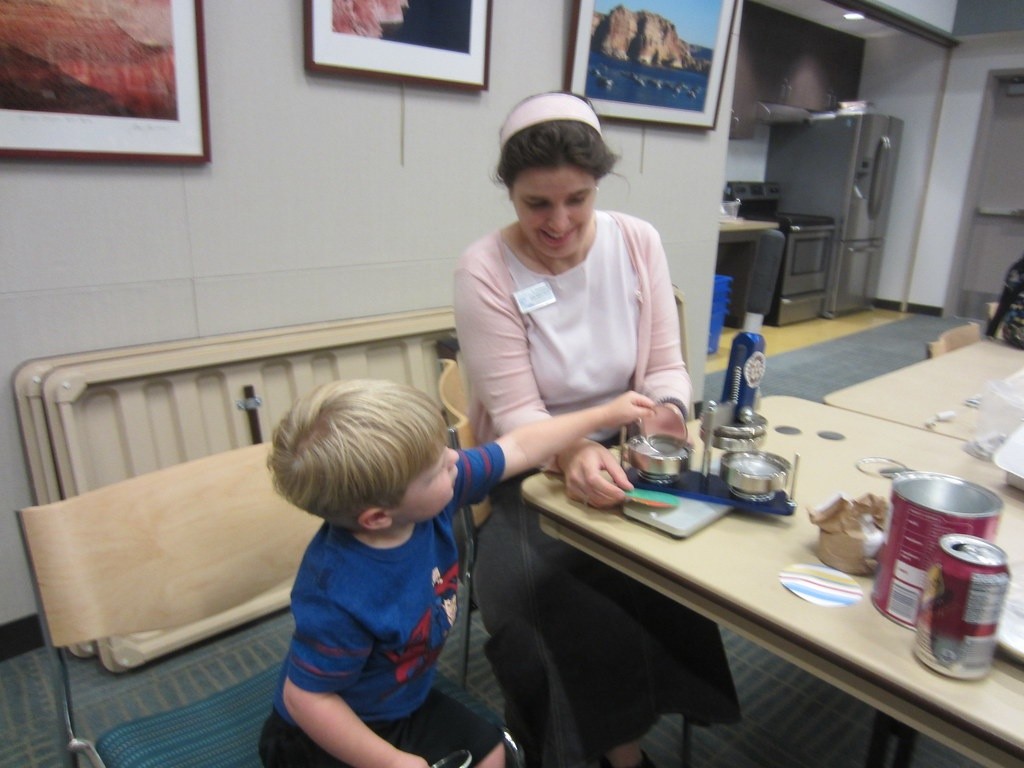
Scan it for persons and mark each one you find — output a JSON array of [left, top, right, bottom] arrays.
[[454, 92, 739, 768], [260, 379, 657, 768]]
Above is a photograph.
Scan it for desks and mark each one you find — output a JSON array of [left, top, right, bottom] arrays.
[[522, 394, 1024, 768], [824, 340, 1024, 442], [718, 220, 780, 328]]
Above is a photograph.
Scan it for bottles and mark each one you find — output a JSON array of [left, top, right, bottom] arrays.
[[724, 312, 767, 412]]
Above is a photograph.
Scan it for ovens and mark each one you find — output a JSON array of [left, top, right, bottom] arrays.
[[768, 223, 834, 326]]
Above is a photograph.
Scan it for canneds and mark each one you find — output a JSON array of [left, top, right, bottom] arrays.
[[872, 469, 1004, 633], [913, 534, 1011, 680]]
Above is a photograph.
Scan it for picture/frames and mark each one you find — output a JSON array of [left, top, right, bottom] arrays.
[[564, 0, 737, 131], [0, 0, 213, 164], [304, 0, 494, 90]]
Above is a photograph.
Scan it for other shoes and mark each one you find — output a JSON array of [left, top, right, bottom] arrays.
[[602, 748, 656, 768]]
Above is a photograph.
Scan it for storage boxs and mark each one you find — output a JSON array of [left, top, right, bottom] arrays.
[[708, 275, 733, 354]]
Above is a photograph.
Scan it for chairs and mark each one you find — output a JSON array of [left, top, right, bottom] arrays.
[[15, 440, 325, 768], [926, 321, 980, 359], [437, 356, 692, 768]]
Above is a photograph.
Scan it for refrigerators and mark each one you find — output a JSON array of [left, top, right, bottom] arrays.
[[764, 110, 905, 319]]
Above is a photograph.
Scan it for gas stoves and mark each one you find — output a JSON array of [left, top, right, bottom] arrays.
[[728, 180, 835, 226]]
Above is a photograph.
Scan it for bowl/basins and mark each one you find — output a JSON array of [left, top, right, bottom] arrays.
[[629, 435, 693, 478], [720, 200, 740, 223], [721, 449, 791, 496]]
